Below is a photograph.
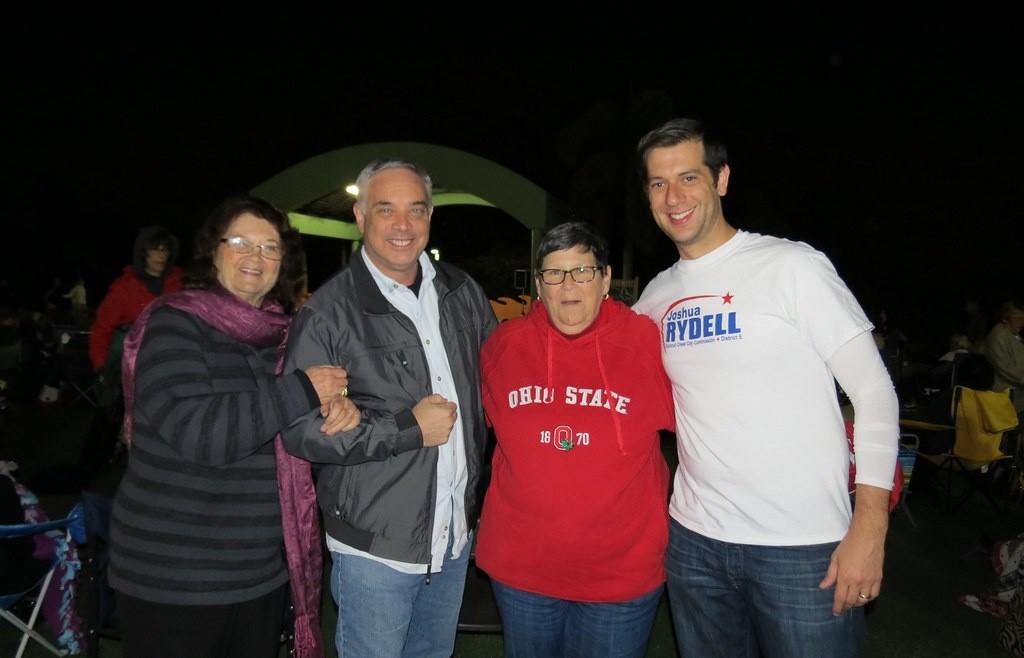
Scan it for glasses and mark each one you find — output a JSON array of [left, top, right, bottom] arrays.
[[148, 245, 173, 256], [218, 235, 288, 261], [538, 266, 602, 285]]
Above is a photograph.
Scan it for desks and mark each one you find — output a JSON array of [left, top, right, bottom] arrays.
[[40, 325, 101, 413]]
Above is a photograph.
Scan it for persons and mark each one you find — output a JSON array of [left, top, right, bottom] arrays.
[[108, 197, 360, 658], [630, 121, 900, 657], [279, 159, 499, 657], [475, 224, 677, 657], [878, 309, 894, 337], [89, 227, 183, 470], [959, 303, 978, 343], [0, 473, 36, 596], [985, 303, 1024, 488], [63, 280, 88, 322]]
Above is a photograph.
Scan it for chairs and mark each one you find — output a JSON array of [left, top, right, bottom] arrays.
[[883, 435, 923, 531], [0, 460, 84, 658], [905, 386, 1020, 524]]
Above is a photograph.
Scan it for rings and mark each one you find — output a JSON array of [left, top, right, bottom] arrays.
[[342, 387, 347, 396], [857, 595, 871, 600]]
